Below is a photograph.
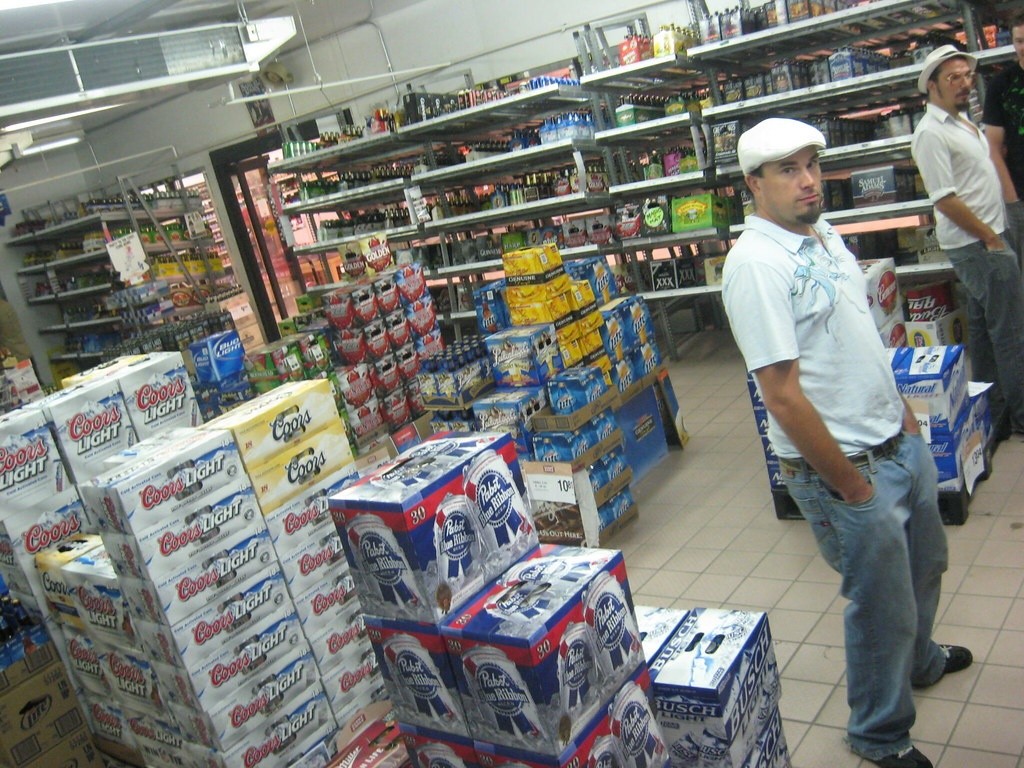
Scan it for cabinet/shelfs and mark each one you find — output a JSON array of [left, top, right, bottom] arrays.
[[4, 175, 245, 363], [265, 0, 991, 361]]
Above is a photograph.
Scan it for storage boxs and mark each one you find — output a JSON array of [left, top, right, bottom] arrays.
[[0, 165, 993, 768]]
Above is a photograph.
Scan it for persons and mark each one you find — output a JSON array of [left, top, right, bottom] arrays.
[[911, 19, 1024, 437], [722, 118, 974, 768]]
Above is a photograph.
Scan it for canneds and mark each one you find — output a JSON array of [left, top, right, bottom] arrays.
[[345, 450, 671, 768], [281, 141, 324, 159]]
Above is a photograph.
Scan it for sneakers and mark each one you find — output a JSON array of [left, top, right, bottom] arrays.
[[869, 746, 935, 768], [940, 644, 973, 673]]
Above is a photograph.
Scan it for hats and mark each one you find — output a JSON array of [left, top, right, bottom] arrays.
[[737, 120, 827, 174], [918, 45, 977, 94]]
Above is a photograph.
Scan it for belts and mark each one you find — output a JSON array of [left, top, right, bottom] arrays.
[[782, 432, 904, 469]]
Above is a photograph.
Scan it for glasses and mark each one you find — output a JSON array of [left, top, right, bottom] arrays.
[[947, 71, 974, 85]]
